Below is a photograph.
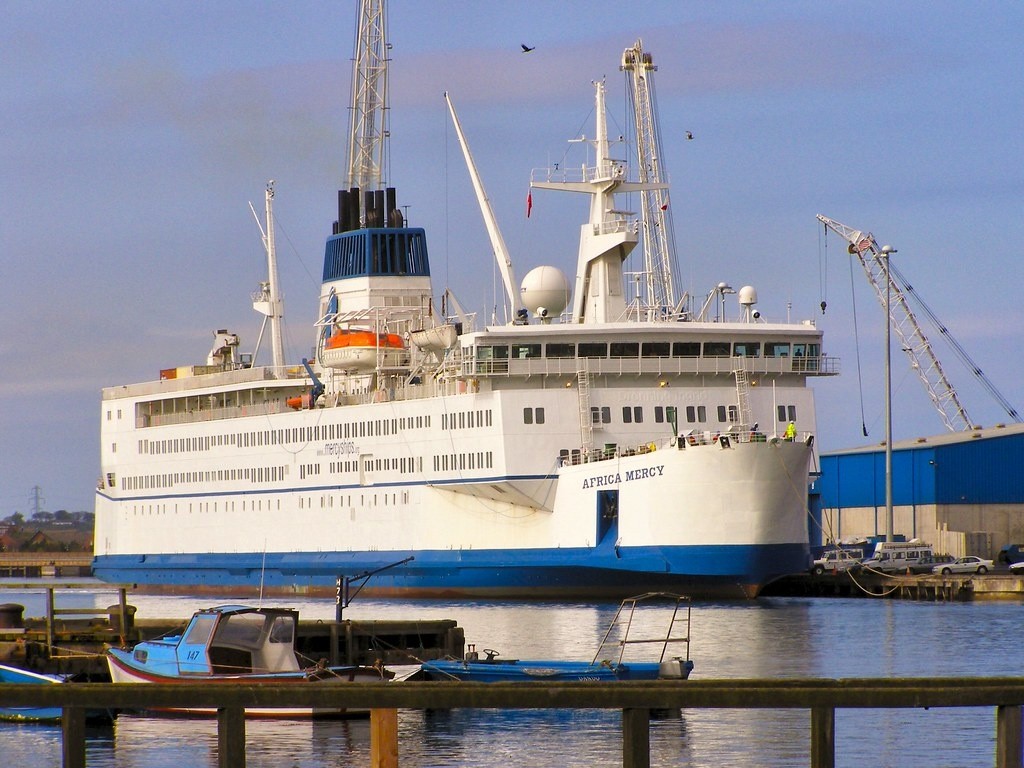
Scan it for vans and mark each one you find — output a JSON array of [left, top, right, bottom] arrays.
[[871, 542, 934, 557], [861, 546, 934, 574]]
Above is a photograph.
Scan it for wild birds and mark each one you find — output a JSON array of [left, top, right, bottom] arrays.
[[521, 44, 536, 53], [685, 130, 695, 140]]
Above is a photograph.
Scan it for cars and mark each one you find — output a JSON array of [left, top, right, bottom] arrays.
[[1009, 561, 1024, 575], [897, 554, 956, 575], [932, 556, 995, 576]]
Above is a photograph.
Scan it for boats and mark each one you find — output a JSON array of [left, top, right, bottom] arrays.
[[0, 665, 105, 723], [422, 591, 695, 679], [101, 601, 398, 721]]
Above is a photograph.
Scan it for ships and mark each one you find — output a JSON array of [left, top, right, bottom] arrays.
[[89, 0, 832, 598]]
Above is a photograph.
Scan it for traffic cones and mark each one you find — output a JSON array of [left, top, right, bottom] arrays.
[[906, 565, 911, 577], [832, 565, 838, 575]]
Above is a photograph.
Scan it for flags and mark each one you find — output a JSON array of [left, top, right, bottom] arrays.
[[527, 187, 532, 217]]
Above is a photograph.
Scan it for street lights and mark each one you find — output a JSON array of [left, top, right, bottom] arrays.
[[883, 243, 895, 546]]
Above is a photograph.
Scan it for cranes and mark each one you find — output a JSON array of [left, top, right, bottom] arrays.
[[812, 207, 1024, 434]]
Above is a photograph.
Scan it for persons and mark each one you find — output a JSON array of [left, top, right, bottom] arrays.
[[788, 420, 798, 441], [750, 422, 758, 433], [562, 433, 695, 467], [713, 430, 720, 440]]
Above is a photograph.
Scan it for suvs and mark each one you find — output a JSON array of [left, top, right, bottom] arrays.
[[812, 548, 865, 575]]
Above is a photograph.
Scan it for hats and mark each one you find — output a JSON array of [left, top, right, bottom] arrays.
[[755, 422, 759, 425], [790, 421, 794, 424]]
